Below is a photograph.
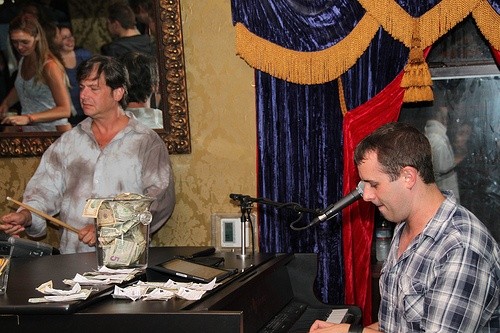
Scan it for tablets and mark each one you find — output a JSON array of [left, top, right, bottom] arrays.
[[149, 255, 235, 283]]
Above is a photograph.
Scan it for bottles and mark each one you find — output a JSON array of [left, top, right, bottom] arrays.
[[375, 222, 392, 261]]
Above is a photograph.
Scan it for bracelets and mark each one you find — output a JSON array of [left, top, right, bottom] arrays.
[[348, 324, 364, 333], [27, 114, 32, 122]]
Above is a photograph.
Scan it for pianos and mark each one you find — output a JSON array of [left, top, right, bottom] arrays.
[[0, 246, 363, 333]]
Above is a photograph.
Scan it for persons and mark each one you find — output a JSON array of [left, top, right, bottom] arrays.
[[309, 122, 500, 333], [45, 21, 93, 125], [118, 51, 163, 129], [100, 3, 157, 106], [0, 5, 71, 132], [0, 55, 175, 254]]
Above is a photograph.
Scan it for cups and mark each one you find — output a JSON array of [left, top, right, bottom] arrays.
[[0, 255, 12, 294], [85, 196, 156, 273]]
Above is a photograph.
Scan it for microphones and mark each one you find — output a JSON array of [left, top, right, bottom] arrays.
[[310, 181, 365, 227]]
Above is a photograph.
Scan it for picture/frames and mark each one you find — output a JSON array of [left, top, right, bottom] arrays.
[[0, 0, 192, 158]]
